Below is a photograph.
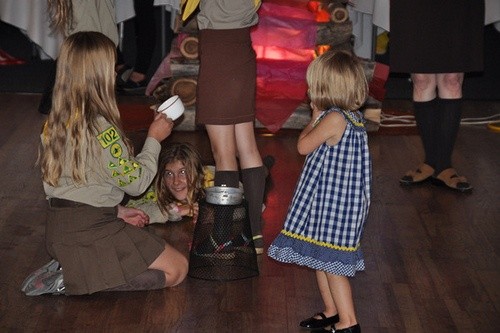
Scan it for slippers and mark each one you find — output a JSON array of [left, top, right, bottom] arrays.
[[398, 162, 435, 185], [433, 167, 474, 192]]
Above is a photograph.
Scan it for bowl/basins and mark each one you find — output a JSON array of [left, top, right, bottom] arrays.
[[205, 184, 245, 205]]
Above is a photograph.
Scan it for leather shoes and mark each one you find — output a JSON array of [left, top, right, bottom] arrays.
[[299, 312, 362, 333]]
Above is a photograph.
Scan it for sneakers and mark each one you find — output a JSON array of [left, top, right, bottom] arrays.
[[234, 232, 265, 253], [193, 231, 236, 258]]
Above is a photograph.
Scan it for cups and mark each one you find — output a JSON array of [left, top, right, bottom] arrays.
[[156, 94, 185, 121]]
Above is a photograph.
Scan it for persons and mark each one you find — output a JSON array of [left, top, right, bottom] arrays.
[[386, 0, 488, 194], [194, 0, 266, 255], [267, 47, 374, 333], [118, 126, 275, 225], [0, 0, 135, 113], [19, 29, 189, 298]]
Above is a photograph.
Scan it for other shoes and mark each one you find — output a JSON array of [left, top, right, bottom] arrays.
[[20, 259, 71, 297], [122, 77, 148, 91]]
